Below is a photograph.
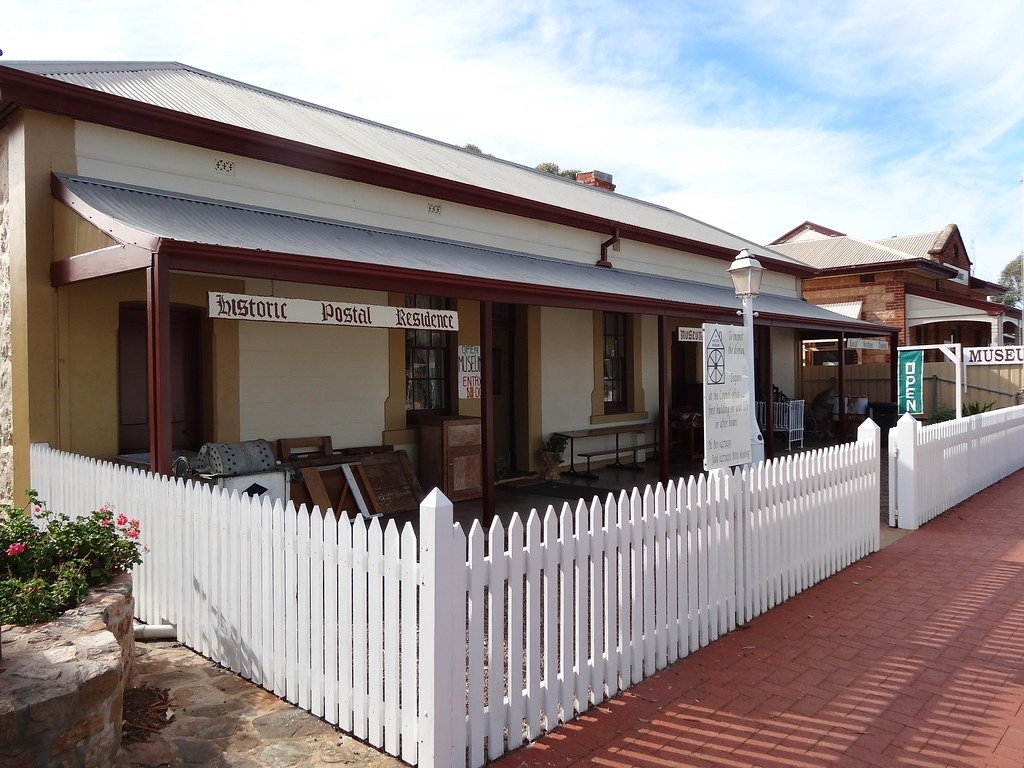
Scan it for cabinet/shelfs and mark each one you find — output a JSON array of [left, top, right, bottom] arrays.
[[417, 414, 483, 502]]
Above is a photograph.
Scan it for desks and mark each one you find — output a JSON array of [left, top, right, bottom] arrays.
[[552, 423, 660, 476], [667, 421, 703, 461]]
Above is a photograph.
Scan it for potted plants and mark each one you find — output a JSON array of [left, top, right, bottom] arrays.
[[539, 432, 569, 480]]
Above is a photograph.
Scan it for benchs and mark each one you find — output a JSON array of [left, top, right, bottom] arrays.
[[577, 442, 660, 481]]
